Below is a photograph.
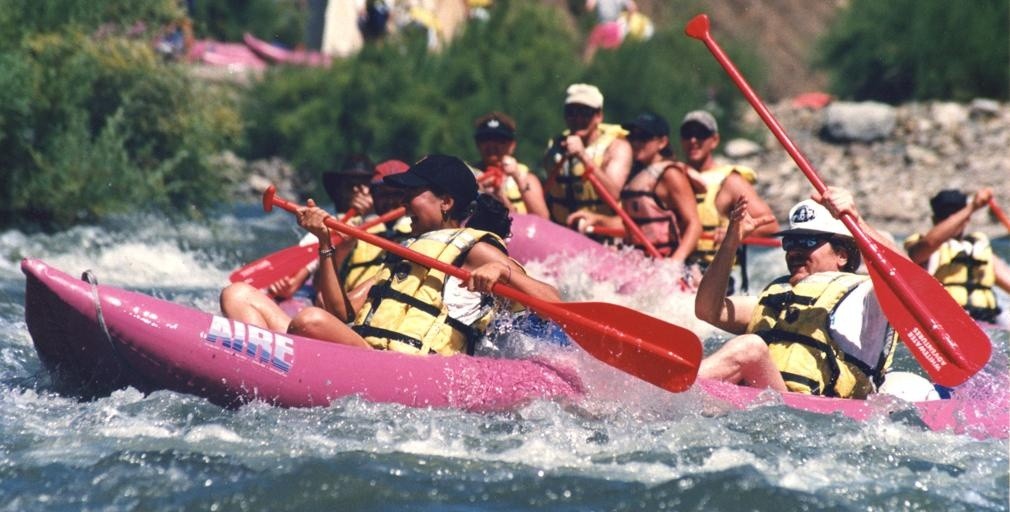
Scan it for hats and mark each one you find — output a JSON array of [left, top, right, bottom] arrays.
[[565, 84, 719, 135], [931, 190, 965, 213], [372, 114, 516, 206], [775, 199, 853, 237]]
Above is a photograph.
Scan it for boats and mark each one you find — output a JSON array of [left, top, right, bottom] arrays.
[[19, 255, 1008, 450], [458, 207, 1005, 358], [85, 20, 268, 90], [240, 26, 337, 74]]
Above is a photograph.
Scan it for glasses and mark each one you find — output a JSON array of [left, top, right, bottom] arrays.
[[782, 238, 830, 248]]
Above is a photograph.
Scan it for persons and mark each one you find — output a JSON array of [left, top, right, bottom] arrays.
[[695, 194, 914, 401], [221, 152, 561, 357], [903, 189, 1010, 326], [545, 83, 634, 251], [680, 111, 780, 297], [474, 113, 550, 222], [358, 1, 654, 63], [567, 113, 703, 283], [158, 14, 183, 56]]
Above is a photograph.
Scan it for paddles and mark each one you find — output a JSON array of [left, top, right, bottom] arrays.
[[263, 186, 702, 393], [229, 167, 504, 289], [685, 14, 991, 389]]
